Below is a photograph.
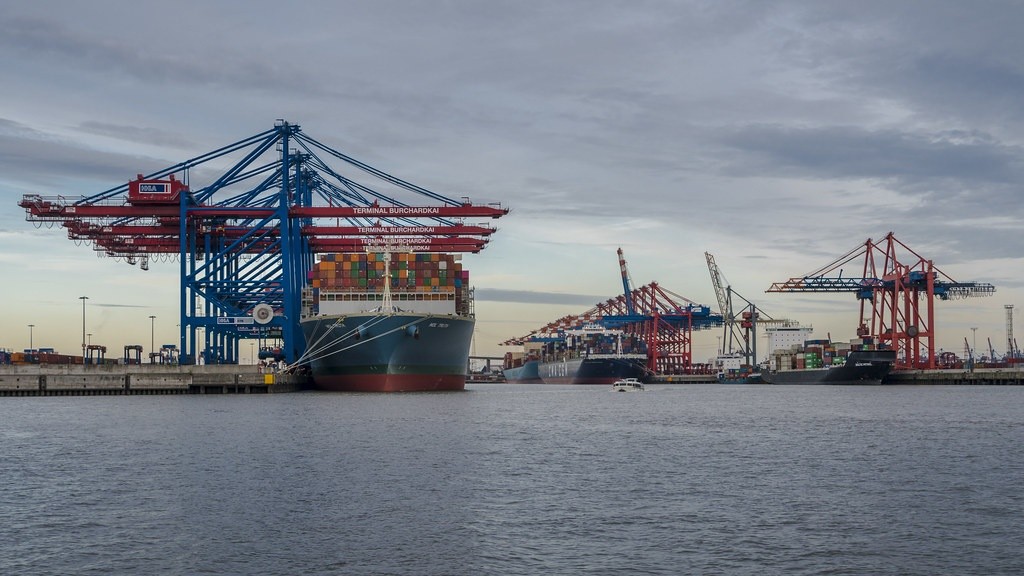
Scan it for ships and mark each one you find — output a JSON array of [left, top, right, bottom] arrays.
[[760, 350, 898, 384], [299, 246, 478, 391], [502, 320, 655, 384]]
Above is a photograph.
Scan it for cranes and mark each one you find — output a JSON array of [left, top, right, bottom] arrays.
[[763, 231, 997, 370], [499, 247, 725, 376], [17, 118, 509, 375], [704, 252, 797, 370]]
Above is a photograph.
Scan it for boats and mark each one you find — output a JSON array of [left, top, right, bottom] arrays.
[[612, 378, 645, 393], [712, 367, 762, 384]]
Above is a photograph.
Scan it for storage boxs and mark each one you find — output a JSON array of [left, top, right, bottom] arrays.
[[306, 252, 470, 316], [130, 179, 206, 226], [0, 351, 141, 364], [759, 337, 887, 372], [502, 332, 647, 370]]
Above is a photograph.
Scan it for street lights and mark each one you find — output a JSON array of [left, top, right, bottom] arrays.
[[971, 327, 978, 357], [79, 297, 89, 365], [716, 335, 722, 356], [86, 334, 92, 345], [149, 316, 156, 354], [28, 325, 35, 356], [250, 343, 254, 366]]
